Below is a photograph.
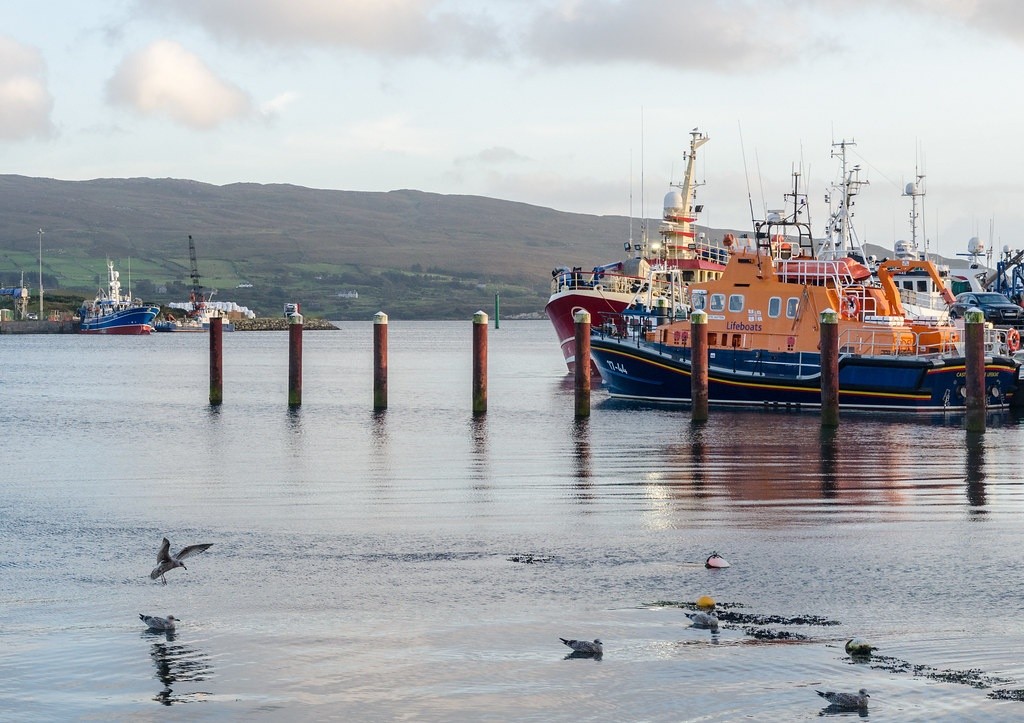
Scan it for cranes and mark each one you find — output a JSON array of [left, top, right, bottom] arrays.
[[188, 235, 205, 314]]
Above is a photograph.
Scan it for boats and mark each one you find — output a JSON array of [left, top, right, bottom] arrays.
[[544, 126, 1024, 416], [77, 273, 161, 334], [154, 320, 208, 332]]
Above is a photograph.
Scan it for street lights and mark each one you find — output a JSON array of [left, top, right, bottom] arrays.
[[37, 228, 45, 320]]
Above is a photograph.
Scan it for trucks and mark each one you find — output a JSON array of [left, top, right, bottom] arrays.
[[284, 303, 299, 318]]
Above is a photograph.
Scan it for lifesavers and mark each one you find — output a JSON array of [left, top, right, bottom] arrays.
[[840, 295, 861, 317], [1007, 328, 1021, 352]]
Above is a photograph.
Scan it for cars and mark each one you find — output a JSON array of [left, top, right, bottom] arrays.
[[948, 292, 1024, 325], [26, 313, 38, 321]]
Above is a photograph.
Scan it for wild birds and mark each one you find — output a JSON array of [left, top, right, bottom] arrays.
[[683, 612, 720, 627], [138, 613, 181, 630], [559, 637, 604, 654], [151, 537, 214, 585], [814, 688, 870, 709]]
